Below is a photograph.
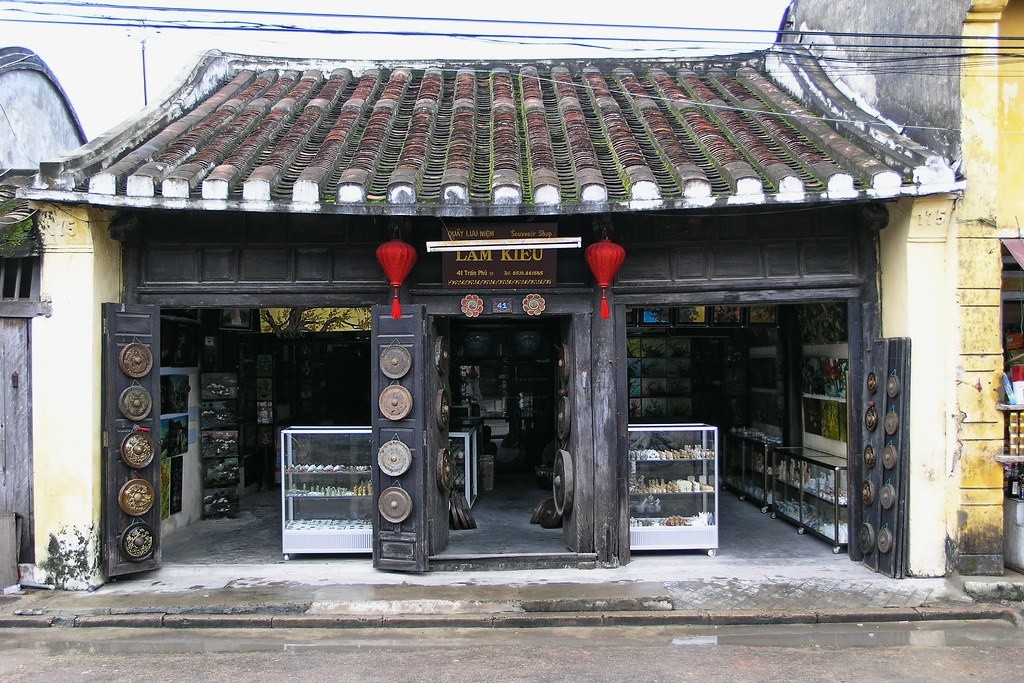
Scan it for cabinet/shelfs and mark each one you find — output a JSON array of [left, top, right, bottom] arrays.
[[771, 446, 849, 554], [628, 423, 719, 557], [448, 427, 478, 509], [280, 426, 375, 560], [720, 432, 780, 513]]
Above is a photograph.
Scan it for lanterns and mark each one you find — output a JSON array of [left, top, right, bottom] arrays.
[[376, 242, 417, 320], [585, 240, 626, 319]]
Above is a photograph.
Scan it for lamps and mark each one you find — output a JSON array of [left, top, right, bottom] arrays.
[[426, 236, 582, 252]]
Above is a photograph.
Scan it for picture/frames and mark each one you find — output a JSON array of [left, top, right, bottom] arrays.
[[159, 309, 203, 325], [218, 308, 254, 332], [624, 302, 778, 327]]
